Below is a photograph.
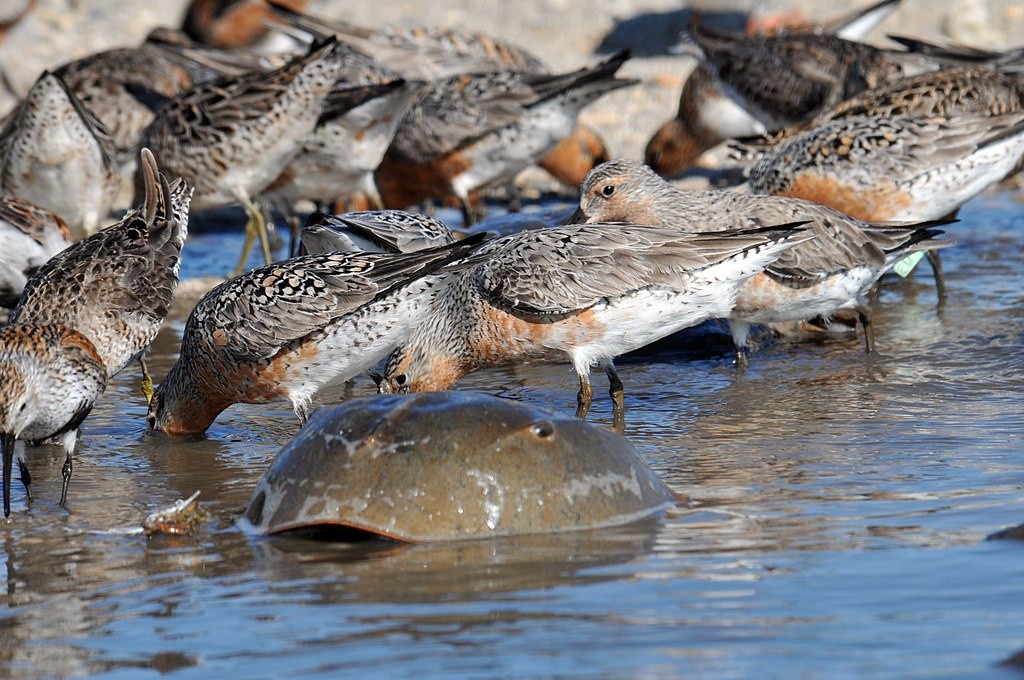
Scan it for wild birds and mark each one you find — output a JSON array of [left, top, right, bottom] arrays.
[[0, 1, 1024, 516]]
